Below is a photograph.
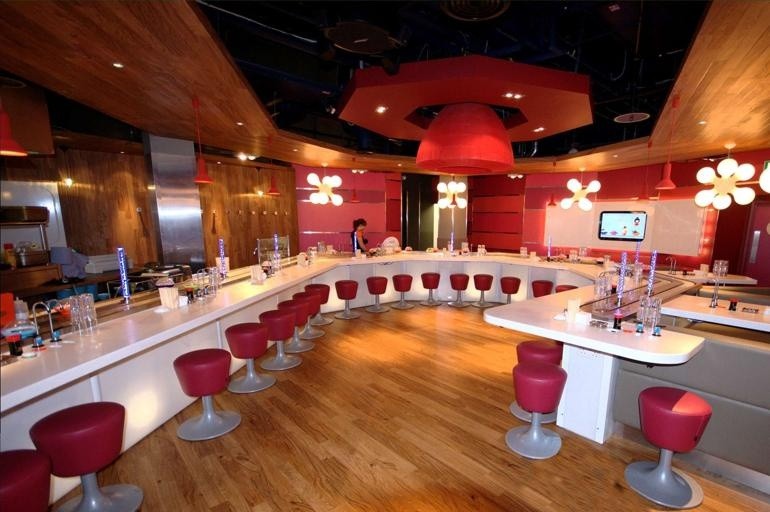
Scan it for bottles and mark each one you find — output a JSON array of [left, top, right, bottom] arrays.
[[186, 267, 218, 303], [463, 242, 469, 256], [447, 241, 451, 252], [4, 243, 17, 269], [729, 298, 738, 311], [13, 297, 29, 322], [612, 295, 622, 330], [477, 244, 487, 256]]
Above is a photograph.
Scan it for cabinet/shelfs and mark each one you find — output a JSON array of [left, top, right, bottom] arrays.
[[1, 206, 64, 289]]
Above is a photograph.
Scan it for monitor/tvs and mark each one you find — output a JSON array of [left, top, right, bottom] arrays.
[[598, 210, 647, 241]]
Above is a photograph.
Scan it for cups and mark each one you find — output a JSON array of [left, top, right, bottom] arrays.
[[568, 299, 578, 319], [6, 333, 23, 357], [356, 249, 361, 259], [530, 252, 536, 261], [310, 242, 333, 263], [570, 250, 577, 263], [579, 247, 586, 257], [713, 260, 729, 278], [73, 320, 95, 336], [700, 264, 709, 274], [590, 300, 609, 328], [636, 263, 643, 276], [0, 292, 16, 326], [637, 294, 661, 323], [519, 246, 527, 257], [362, 254, 366, 260], [69, 293, 97, 323], [593, 275, 612, 299]]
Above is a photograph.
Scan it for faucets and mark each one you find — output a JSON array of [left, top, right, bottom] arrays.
[[196, 269, 203, 284], [665, 256, 671, 274], [708, 279, 717, 309], [201, 267, 212, 288], [46, 299, 63, 343], [714, 277, 719, 306], [31, 302, 52, 348], [670, 256, 677, 275]]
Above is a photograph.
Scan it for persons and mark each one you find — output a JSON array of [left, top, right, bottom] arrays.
[[351, 217, 374, 256]]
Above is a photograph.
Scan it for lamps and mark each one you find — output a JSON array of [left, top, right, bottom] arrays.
[[558, 168, 604, 214], [264, 135, 283, 197], [547, 158, 557, 208], [306, 161, 347, 207], [436, 172, 471, 212], [187, 94, 214, 184], [690, 142, 759, 213], [0, 88, 32, 159], [637, 139, 654, 201], [350, 156, 361, 204], [655, 94, 682, 190]]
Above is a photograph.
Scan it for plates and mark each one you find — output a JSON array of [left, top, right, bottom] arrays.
[[3, 326, 39, 339]]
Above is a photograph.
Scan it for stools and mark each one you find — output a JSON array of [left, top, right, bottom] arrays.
[[0, 247, 720, 511]]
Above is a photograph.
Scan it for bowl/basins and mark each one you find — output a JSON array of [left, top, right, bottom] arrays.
[[98, 292, 109, 300], [15, 250, 49, 267]]
